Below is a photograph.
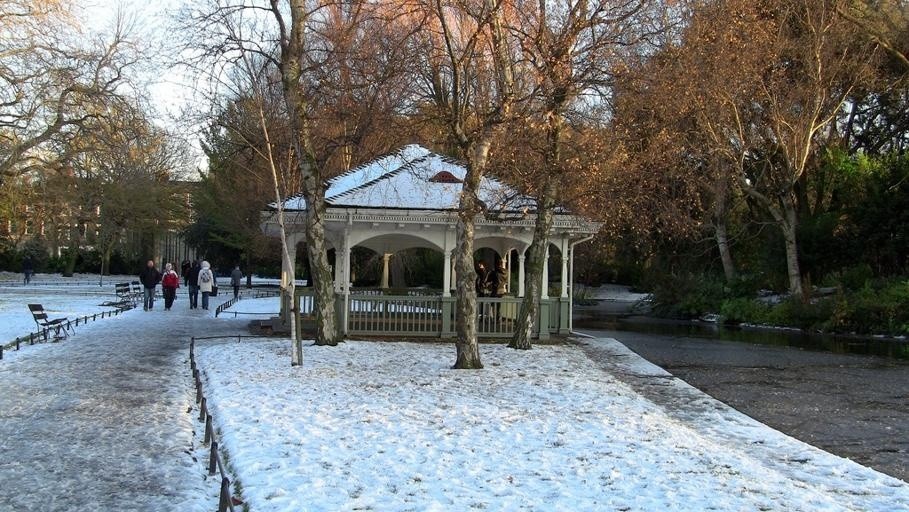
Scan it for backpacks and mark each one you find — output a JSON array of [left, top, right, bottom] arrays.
[[200, 269, 211, 284], [163, 270, 178, 288]]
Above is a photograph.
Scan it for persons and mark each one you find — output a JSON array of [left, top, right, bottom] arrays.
[[160, 266, 167, 299], [486, 259, 509, 324], [184, 260, 191, 279], [23, 255, 32, 286], [230, 264, 242, 298], [140, 260, 161, 312], [161, 263, 178, 312], [154, 255, 160, 272], [476, 258, 492, 324], [172, 263, 178, 276], [197, 261, 214, 311], [184, 262, 198, 310]]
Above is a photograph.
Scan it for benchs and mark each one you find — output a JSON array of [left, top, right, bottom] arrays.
[[115, 280, 161, 308], [27, 303, 76, 341]]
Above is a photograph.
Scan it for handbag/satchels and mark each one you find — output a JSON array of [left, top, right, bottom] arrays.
[[207, 286, 218, 297]]
